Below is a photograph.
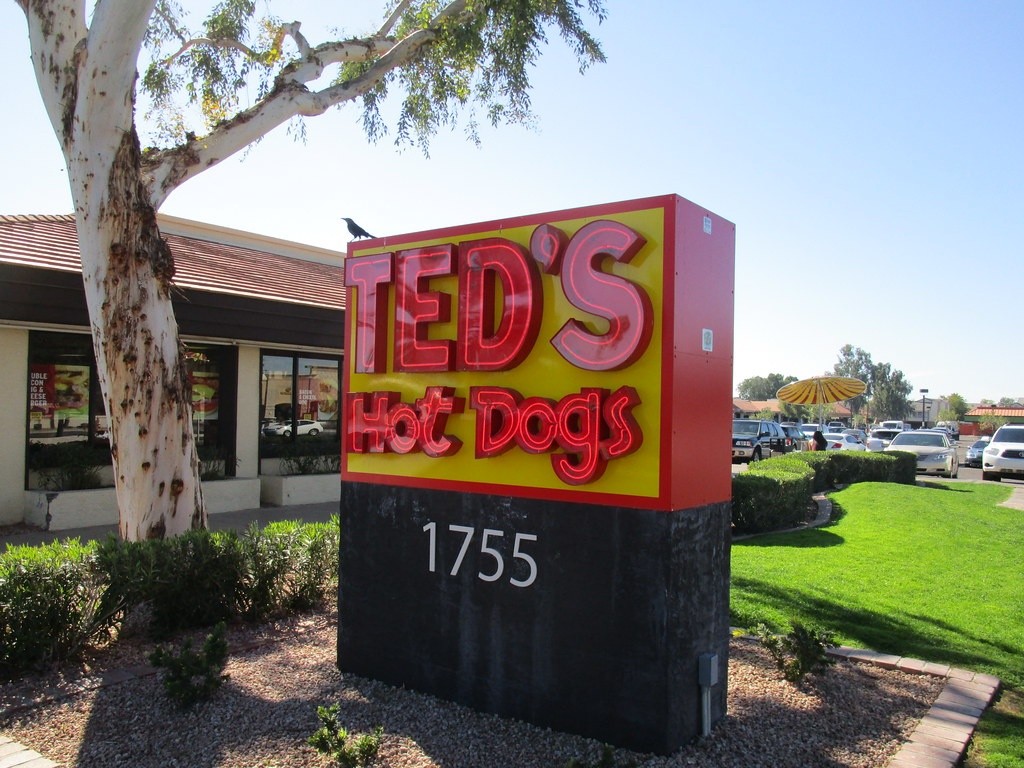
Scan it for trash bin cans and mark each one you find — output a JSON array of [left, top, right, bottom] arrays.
[[769, 437, 794, 457]]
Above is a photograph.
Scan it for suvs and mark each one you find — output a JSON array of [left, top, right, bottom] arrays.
[[732, 417, 787, 466]]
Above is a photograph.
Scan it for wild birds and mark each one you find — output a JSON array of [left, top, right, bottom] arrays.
[[341, 217, 378, 244]]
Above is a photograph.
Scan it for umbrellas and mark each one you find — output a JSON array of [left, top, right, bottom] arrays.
[[776, 376, 867, 431]]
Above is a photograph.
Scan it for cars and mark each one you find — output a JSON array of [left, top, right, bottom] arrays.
[[262, 419, 324, 437], [980, 423, 1024, 481], [964, 440, 989, 468], [779, 420, 959, 452], [880, 431, 960, 480]]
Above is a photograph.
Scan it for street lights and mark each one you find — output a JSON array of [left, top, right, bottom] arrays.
[[305, 364, 313, 414], [262, 373, 270, 406], [920, 388, 929, 428]]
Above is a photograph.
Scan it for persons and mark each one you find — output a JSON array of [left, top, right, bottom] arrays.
[[811, 431, 828, 451]]
